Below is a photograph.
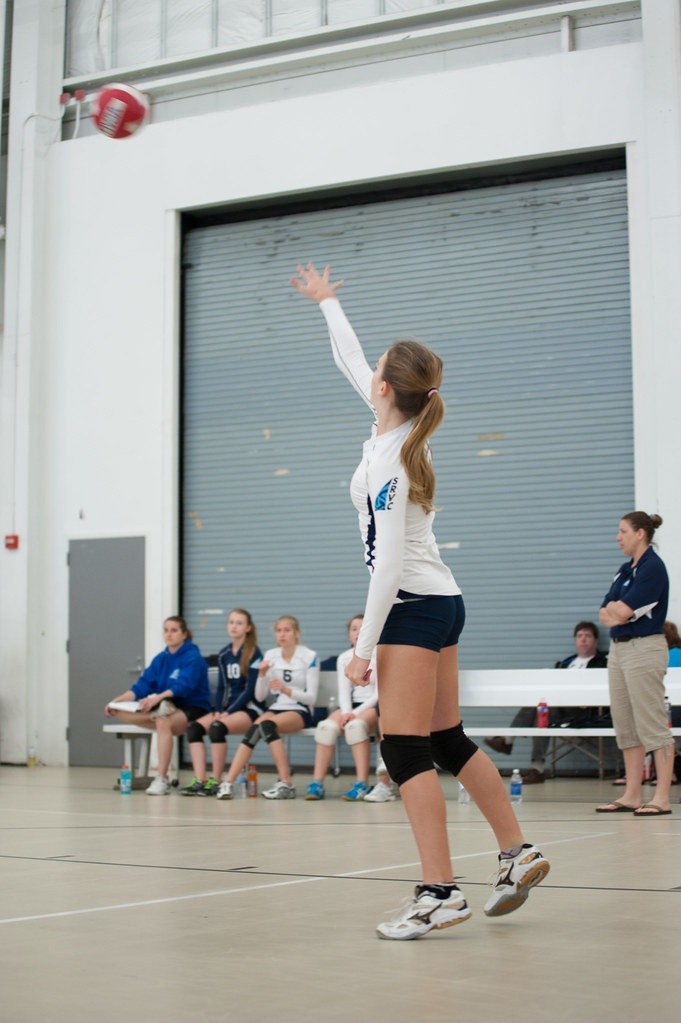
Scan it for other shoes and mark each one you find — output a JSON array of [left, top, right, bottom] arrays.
[[651, 778, 678, 786], [522, 767, 545, 784], [612, 776, 645, 785], [483, 735, 513, 755]]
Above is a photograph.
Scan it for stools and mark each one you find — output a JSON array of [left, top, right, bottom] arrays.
[[550, 707, 610, 781]]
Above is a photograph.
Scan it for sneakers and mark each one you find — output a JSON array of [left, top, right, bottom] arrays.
[[197, 777, 222, 795], [304, 781, 324, 799], [145, 776, 172, 795], [179, 778, 207, 795], [375, 885, 472, 941], [484, 844, 549, 917], [341, 781, 370, 801], [261, 781, 295, 799], [150, 700, 175, 719], [217, 782, 232, 798], [365, 782, 395, 801]]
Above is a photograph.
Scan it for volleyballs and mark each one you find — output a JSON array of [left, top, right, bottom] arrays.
[[90, 82, 148, 140]]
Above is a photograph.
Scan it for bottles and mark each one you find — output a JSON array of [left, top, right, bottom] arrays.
[[459, 781, 470, 806], [247, 765, 257, 796], [537, 696, 548, 728], [120, 765, 132, 794], [327, 696, 336, 715], [237, 768, 247, 797], [509, 769, 523, 804]]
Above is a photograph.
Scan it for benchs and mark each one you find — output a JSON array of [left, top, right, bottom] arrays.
[[103, 674, 339, 783], [457, 666, 680, 738]]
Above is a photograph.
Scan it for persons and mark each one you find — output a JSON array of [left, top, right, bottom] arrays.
[[104, 616, 211, 795], [305, 614, 378, 801], [612, 621, 681, 786], [216, 616, 319, 799], [176, 608, 266, 796], [362, 745, 397, 803], [596, 511, 675, 816], [482, 622, 610, 785], [289, 261, 552, 943]]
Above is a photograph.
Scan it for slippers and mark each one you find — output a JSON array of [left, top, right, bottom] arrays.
[[634, 805, 672, 815], [596, 801, 640, 812]]
